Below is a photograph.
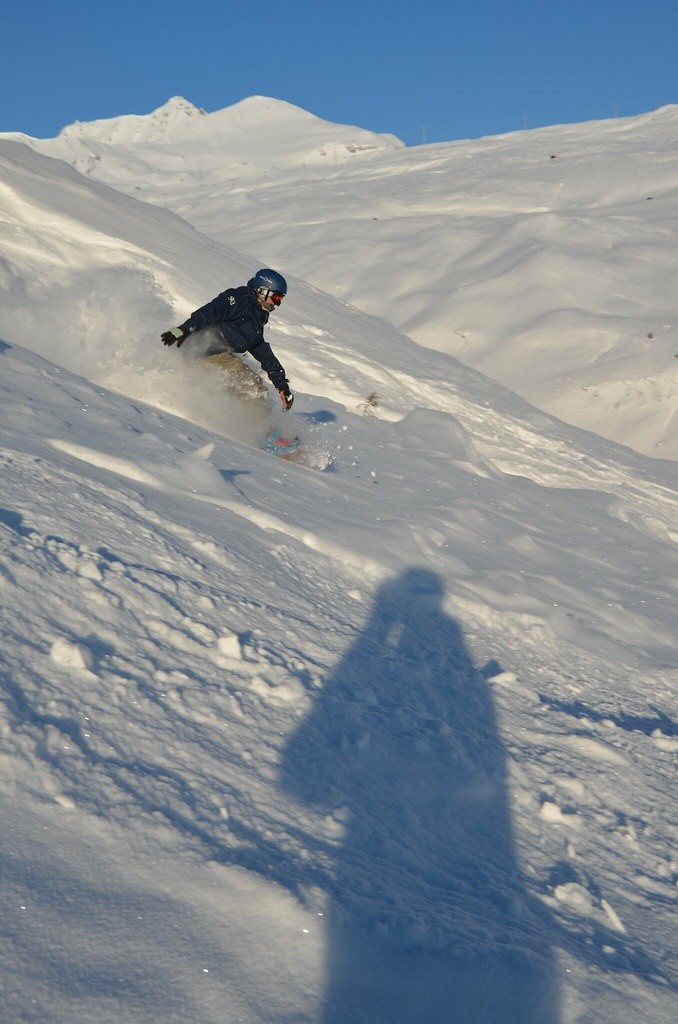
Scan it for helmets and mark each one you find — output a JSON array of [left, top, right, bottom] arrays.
[[247, 269, 287, 312]]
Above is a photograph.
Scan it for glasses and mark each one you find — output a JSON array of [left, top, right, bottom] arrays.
[[258, 288, 284, 306]]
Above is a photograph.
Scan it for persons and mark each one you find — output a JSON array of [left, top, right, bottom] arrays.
[[161, 268, 295, 455]]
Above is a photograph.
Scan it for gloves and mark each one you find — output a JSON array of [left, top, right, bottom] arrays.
[[161, 325, 191, 348], [278, 383, 294, 412]]
